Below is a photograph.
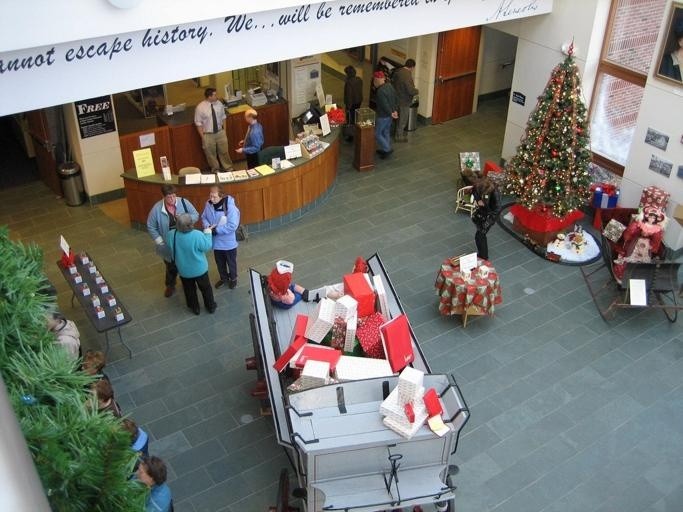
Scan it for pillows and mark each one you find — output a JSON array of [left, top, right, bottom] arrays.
[[601, 218, 626, 244]]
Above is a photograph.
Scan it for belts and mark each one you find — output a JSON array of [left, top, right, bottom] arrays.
[[202, 127, 224, 135]]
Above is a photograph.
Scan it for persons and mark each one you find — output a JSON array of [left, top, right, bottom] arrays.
[[146, 185, 199, 297], [343, 65, 364, 142], [371, 71, 399, 159], [265, 260, 309, 309], [613, 205, 664, 282], [194, 88, 235, 173], [471, 178, 502, 260], [165, 213, 217, 315], [392, 59, 419, 143], [659, 36, 683, 81], [201, 185, 241, 289], [47, 312, 174, 512], [235, 109, 264, 168]]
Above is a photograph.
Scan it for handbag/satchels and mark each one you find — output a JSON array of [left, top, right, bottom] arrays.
[[234, 222, 250, 242]]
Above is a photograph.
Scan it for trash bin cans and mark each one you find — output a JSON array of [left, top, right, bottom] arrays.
[[58, 161, 85, 207], [407, 100, 419, 131]]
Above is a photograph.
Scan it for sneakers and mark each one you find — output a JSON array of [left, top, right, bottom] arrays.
[[376, 149, 394, 160]]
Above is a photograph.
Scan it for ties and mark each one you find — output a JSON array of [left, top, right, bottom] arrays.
[[243, 125, 252, 147], [210, 103, 220, 134]]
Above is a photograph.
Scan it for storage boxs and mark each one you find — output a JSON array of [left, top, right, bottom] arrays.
[[591, 184, 620, 209], [592, 205, 622, 229], [272, 269, 444, 443], [638, 185, 670, 212]]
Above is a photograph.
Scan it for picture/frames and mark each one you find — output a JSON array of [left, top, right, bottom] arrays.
[[652, 0, 682, 88]]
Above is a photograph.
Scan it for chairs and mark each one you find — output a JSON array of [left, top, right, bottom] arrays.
[[454, 185, 477, 217]]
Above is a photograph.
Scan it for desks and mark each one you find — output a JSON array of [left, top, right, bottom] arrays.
[[431, 255, 503, 329], [56, 251, 133, 361]]
